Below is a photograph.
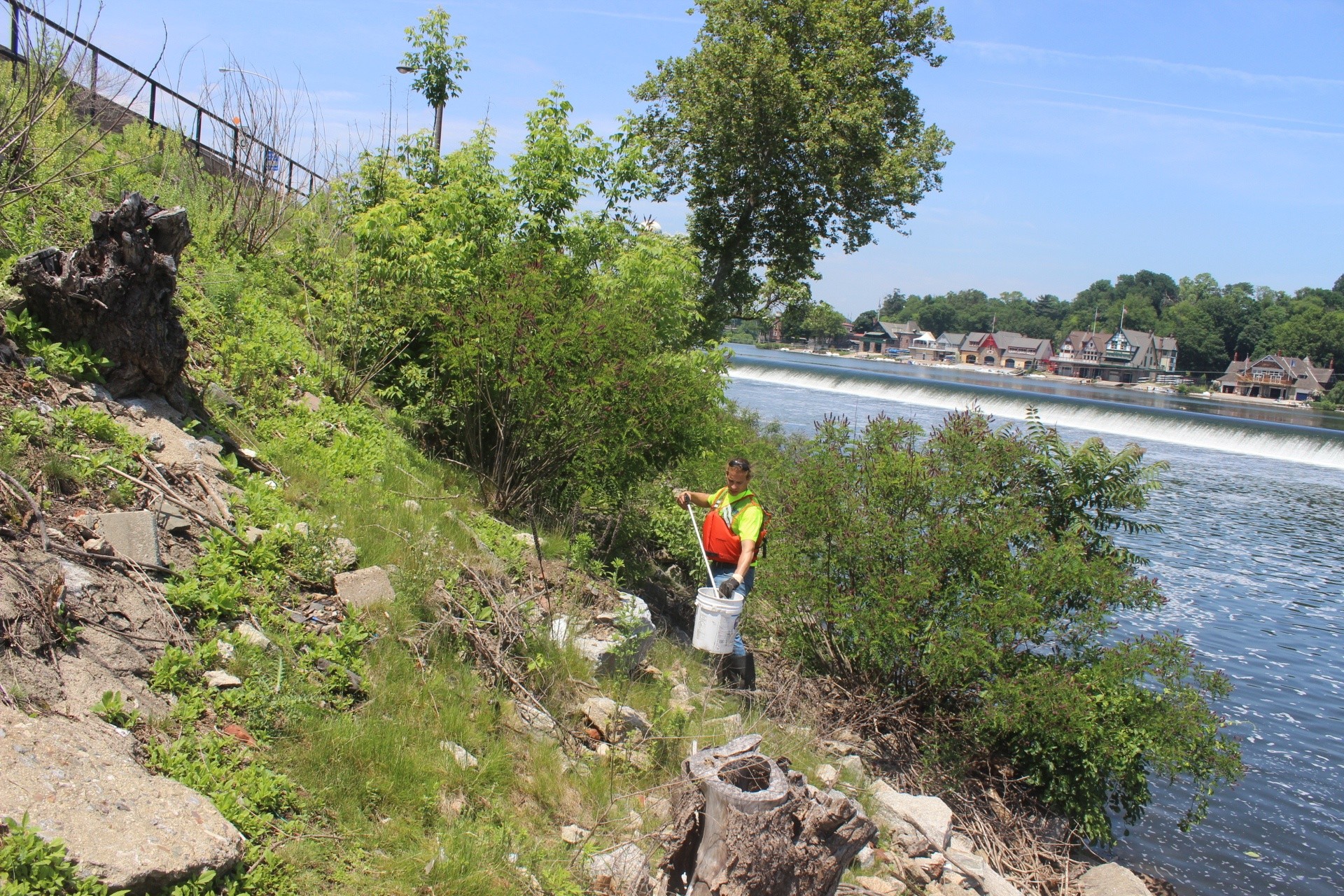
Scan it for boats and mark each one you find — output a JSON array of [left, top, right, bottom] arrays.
[[1139, 386, 1186, 395], [1078, 379, 1096, 385], [907, 360, 940, 368], [1007, 366, 1025, 376], [1334, 407, 1344, 413], [1272, 397, 1299, 407], [799, 349, 839, 357], [780, 347, 789, 352], [975, 367, 1004, 375]]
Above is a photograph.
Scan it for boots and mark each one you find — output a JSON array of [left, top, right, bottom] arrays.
[[723, 653, 756, 702]]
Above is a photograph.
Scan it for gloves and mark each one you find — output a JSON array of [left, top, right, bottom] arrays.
[[719, 578, 740, 598]]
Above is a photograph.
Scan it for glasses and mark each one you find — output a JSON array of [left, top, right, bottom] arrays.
[[729, 460, 751, 473]]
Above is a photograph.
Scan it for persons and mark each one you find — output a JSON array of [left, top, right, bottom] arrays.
[[676, 456, 765, 709]]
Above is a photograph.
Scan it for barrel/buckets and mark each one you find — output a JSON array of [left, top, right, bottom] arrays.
[[691, 588, 744, 654]]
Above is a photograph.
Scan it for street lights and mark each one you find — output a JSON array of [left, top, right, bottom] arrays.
[[219, 68, 278, 188], [396, 64, 443, 179]]
